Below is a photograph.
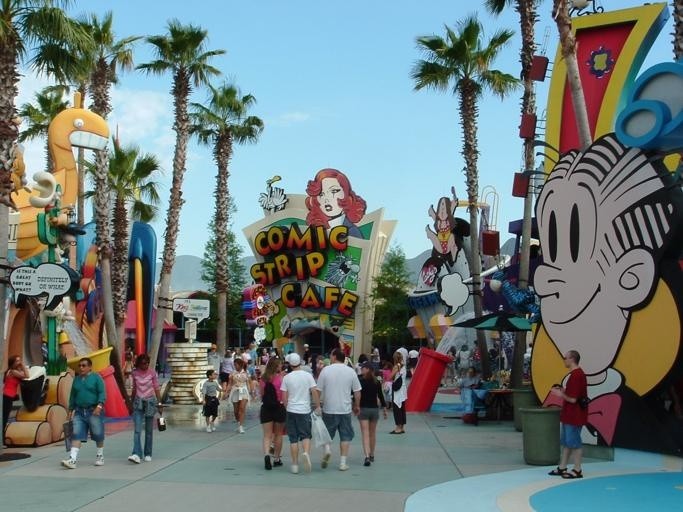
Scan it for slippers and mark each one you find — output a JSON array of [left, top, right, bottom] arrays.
[[390, 430, 400, 434], [401, 430, 406, 434]]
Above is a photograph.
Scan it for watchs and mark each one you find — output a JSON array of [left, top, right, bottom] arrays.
[[158, 403, 162, 405], [97, 405, 103, 409]]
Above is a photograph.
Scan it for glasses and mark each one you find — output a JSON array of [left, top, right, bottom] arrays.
[[78, 364, 90, 367]]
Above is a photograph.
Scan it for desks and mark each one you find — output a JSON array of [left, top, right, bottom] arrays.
[[471, 389, 512, 426]]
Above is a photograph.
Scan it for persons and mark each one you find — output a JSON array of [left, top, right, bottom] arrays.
[[302, 344, 312, 366], [258, 356, 285, 470], [316, 348, 362, 471], [201, 370, 226, 432], [61, 359, 106, 470], [228, 346, 236, 358], [266, 351, 276, 366], [258, 348, 269, 377], [220, 350, 234, 390], [128, 353, 163, 464], [408, 346, 419, 375], [246, 359, 256, 380], [242, 347, 251, 363], [239, 346, 248, 362], [353, 363, 387, 465], [125, 344, 133, 360], [225, 358, 253, 433], [388, 351, 408, 434], [548, 351, 589, 479], [368, 345, 381, 371], [305, 170, 366, 240], [324, 353, 330, 366], [356, 353, 368, 374], [207, 344, 222, 381], [121, 355, 134, 388], [251, 375, 259, 400], [443, 346, 456, 388], [472, 339, 481, 362], [2, 355, 29, 449], [427, 197, 459, 260], [382, 362, 395, 409], [233, 347, 243, 360], [285, 350, 293, 364], [308, 353, 319, 380], [531, 133, 677, 443], [398, 343, 409, 365], [455, 345, 471, 372], [488, 341, 499, 360], [527, 342, 533, 377], [457, 366, 481, 395], [247, 343, 258, 367], [280, 353, 323, 474]]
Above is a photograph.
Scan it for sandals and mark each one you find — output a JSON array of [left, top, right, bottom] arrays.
[[364, 457, 370, 465], [547, 466, 567, 476], [562, 469, 583, 479], [273, 455, 283, 467], [369, 453, 375, 462], [265, 455, 272, 470]]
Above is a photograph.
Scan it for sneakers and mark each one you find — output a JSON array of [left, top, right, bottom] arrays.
[[321, 450, 333, 469], [94, 457, 104, 466], [61, 458, 76, 469], [206, 424, 212, 433], [128, 455, 141, 464], [339, 464, 350, 470], [301, 452, 311, 474], [144, 456, 151, 462], [211, 423, 216, 431], [291, 465, 299, 474], [239, 425, 244, 434]]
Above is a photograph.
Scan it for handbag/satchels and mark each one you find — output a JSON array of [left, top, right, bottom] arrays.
[[158, 417, 167, 432], [382, 382, 393, 402], [578, 396, 591, 407], [63, 417, 73, 451], [393, 366, 403, 391]]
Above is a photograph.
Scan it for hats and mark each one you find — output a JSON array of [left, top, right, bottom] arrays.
[[285, 353, 303, 368], [359, 360, 374, 370]]
